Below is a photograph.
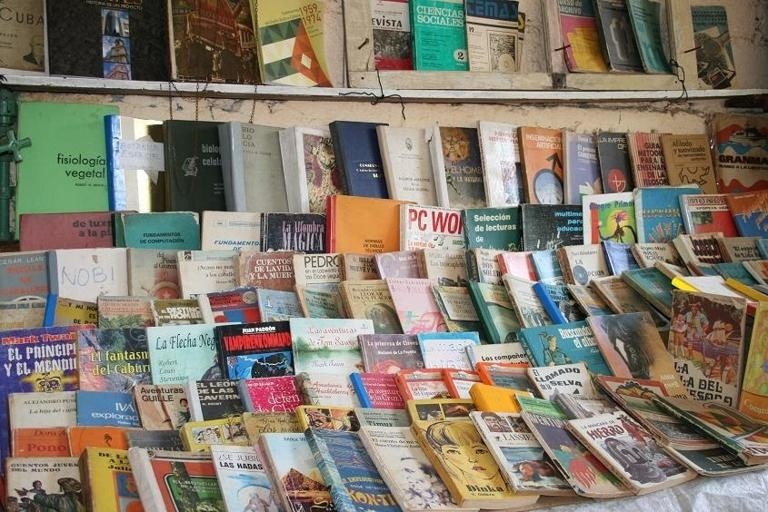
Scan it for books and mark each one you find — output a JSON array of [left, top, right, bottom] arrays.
[[559, 0, 677, 74], [1, 1, 335, 87], [372, 0, 526, 71], [691, 5, 737, 93], [1, 114, 768, 512]]
